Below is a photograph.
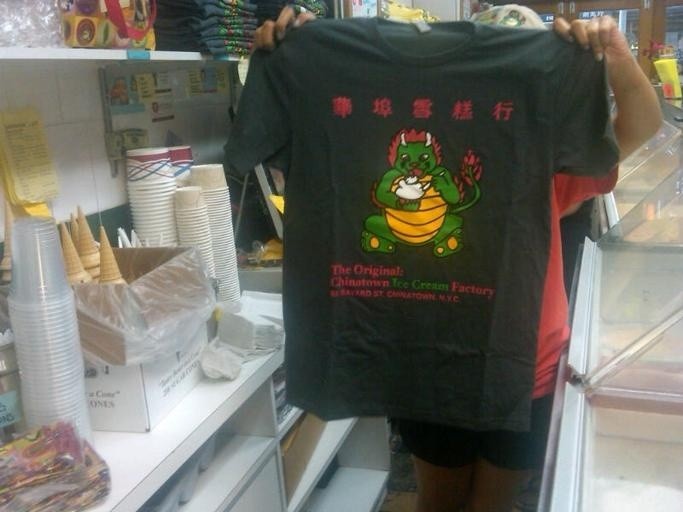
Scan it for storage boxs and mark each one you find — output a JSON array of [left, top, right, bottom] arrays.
[[69, 244, 212, 434]]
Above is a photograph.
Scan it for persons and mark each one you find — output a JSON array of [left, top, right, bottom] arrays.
[[240, 1, 670, 510]]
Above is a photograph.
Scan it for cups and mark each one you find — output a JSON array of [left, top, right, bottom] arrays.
[[125, 143, 242, 314], [58, 208, 129, 285], [9, 219, 88, 448]]
